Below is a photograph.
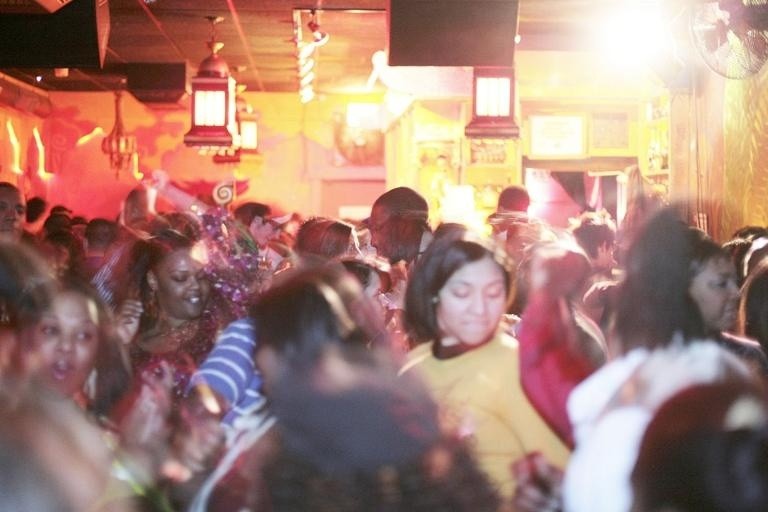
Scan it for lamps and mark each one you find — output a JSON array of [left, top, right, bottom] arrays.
[[99, 81, 139, 180], [280, 3, 332, 105], [462, 64, 520, 142], [184, 13, 259, 167]]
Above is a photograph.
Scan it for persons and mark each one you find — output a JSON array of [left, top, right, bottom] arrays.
[[0, 177, 767, 511]]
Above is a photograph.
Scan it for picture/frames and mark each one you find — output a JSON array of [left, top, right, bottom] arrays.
[[527, 109, 589, 164]]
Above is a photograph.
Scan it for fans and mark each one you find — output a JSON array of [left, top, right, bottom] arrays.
[[682, 0, 767, 85]]
